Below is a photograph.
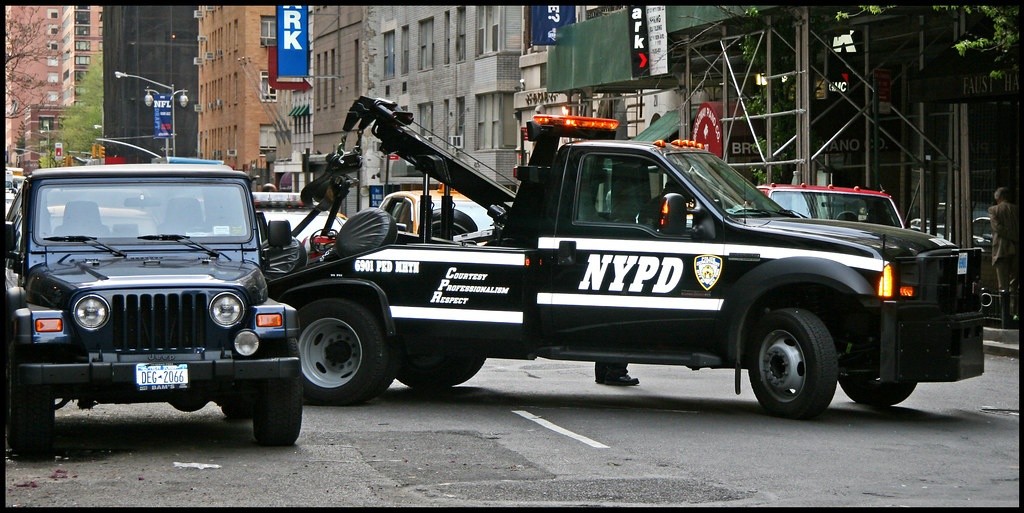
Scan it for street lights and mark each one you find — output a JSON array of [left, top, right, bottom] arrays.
[[115, 71, 189, 157]]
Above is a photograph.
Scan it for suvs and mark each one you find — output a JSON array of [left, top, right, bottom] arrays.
[[6, 160, 306, 460]]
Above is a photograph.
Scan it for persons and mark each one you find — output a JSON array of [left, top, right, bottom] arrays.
[[595, 190, 639, 387], [262, 183, 277, 192], [657, 181, 687, 233], [988, 188, 1018, 314]]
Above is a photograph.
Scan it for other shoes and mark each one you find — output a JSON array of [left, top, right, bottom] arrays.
[[603, 376, 641, 386]]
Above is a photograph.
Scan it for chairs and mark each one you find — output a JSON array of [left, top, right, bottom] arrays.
[[53, 200, 109, 236], [577, 178, 606, 223], [610, 166, 646, 224], [156, 198, 212, 234]]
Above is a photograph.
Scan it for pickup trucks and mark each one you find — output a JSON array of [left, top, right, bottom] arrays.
[[267, 113, 987, 419]]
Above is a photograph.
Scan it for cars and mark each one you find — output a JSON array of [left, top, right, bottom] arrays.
[[5, 166, 906, 290]]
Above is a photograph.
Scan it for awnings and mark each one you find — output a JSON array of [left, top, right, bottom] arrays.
[[629, 110, 702, 142]]
